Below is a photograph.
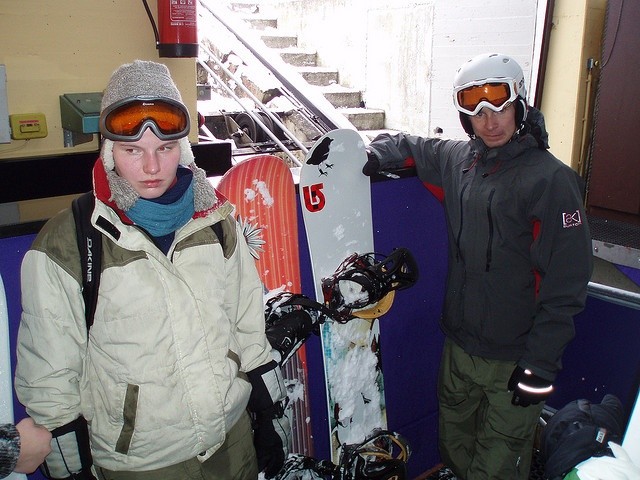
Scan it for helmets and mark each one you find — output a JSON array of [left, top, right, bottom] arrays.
[[453, 54, 528, 134]]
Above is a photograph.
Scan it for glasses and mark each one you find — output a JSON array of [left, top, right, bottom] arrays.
[[99, 95, 190, 141], [453, 77, 524, 116]]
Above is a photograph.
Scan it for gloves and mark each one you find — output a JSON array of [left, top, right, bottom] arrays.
[[362, 149, 380, 176], [538, 394, 625, 476], [253, 424, 284, 479], [507, 367, 553, 407]]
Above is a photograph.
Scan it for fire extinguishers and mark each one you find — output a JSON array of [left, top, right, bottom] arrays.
[[142, 0, 199, 58]]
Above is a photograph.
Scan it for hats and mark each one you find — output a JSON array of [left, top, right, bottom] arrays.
[[100, 61, 194, 174]]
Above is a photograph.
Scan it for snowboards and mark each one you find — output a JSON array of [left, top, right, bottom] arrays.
[[299, 128, 412, 480], [217, 152, 329, 479]]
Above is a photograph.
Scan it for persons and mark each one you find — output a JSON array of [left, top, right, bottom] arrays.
[[0, 416, 52, 478], [13, 60, 285, 480], [363, 55, 594, 479]]
